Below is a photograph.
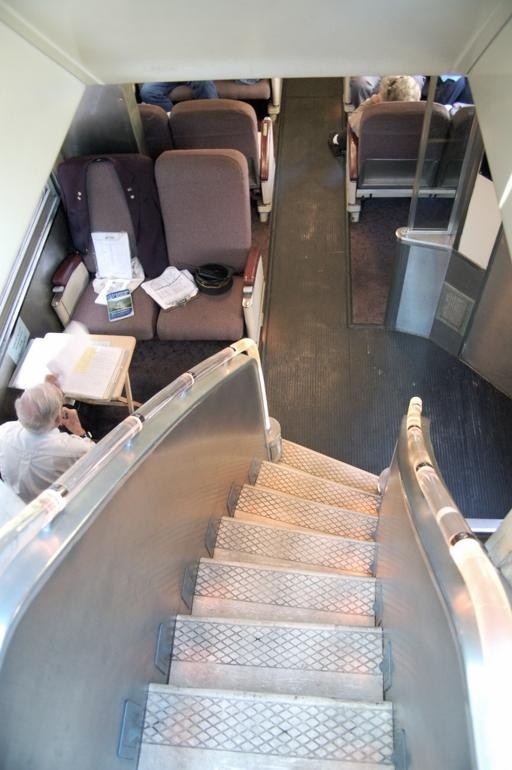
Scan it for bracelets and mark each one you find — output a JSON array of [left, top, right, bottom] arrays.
[[80, 431, 93, 440]]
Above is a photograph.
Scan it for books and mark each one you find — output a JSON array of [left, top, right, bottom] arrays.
[[106, 289, 134, 322]]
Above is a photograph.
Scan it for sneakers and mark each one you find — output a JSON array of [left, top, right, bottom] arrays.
[[328, 131, 342, 157]]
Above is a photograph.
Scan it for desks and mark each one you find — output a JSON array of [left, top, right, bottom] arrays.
[[7, 332, 144, 415]]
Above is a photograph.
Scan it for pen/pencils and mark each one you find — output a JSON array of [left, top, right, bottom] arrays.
[[64, 413, 67, 419]]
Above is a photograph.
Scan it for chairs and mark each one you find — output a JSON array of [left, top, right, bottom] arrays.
[[343, 77, 488, 224]]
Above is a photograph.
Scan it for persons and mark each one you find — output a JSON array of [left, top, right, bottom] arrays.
[[328, 75, 473, 157], [0, 382, 96, 505], [140, 80, 218, 112]]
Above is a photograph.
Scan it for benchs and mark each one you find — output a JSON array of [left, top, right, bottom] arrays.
[[51, 149, 266, 348], [137, 99, 278, 226], [138, 78, 279, 123]]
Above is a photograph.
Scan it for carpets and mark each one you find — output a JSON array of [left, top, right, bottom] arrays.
[[343, 199, 455, 330], [58, 353, 264, 439]]
